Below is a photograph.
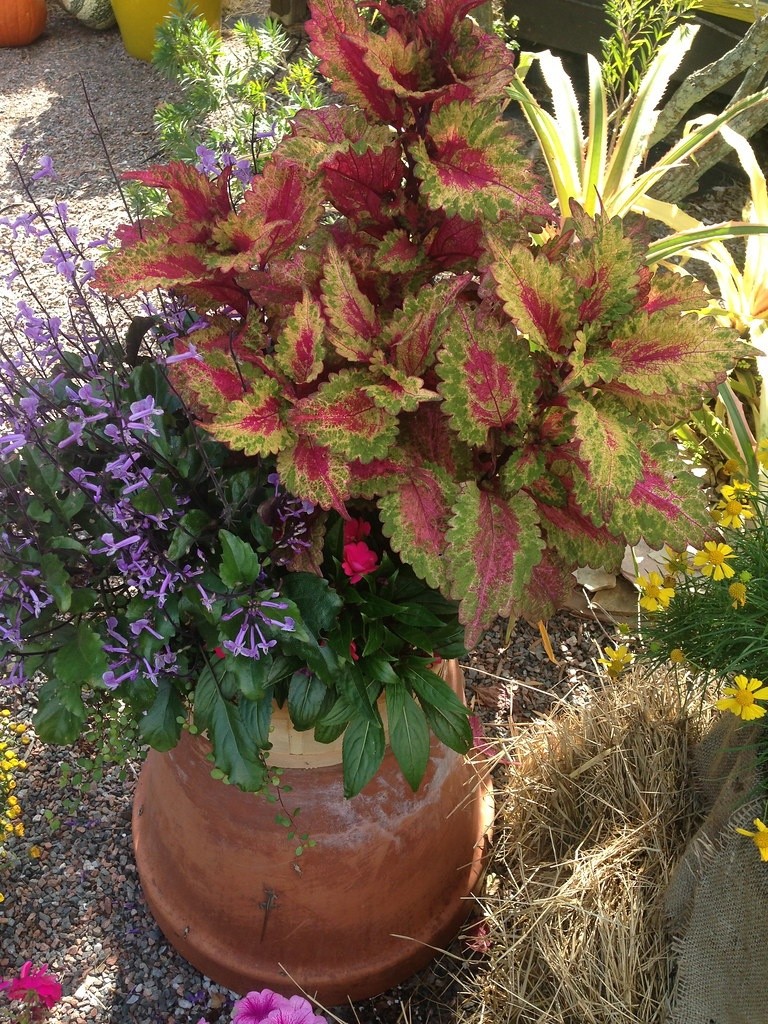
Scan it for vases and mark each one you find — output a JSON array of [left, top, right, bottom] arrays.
[[133, 652, 495, 1008]]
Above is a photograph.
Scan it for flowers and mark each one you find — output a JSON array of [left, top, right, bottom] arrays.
[[0, 0, 768, 864]]
